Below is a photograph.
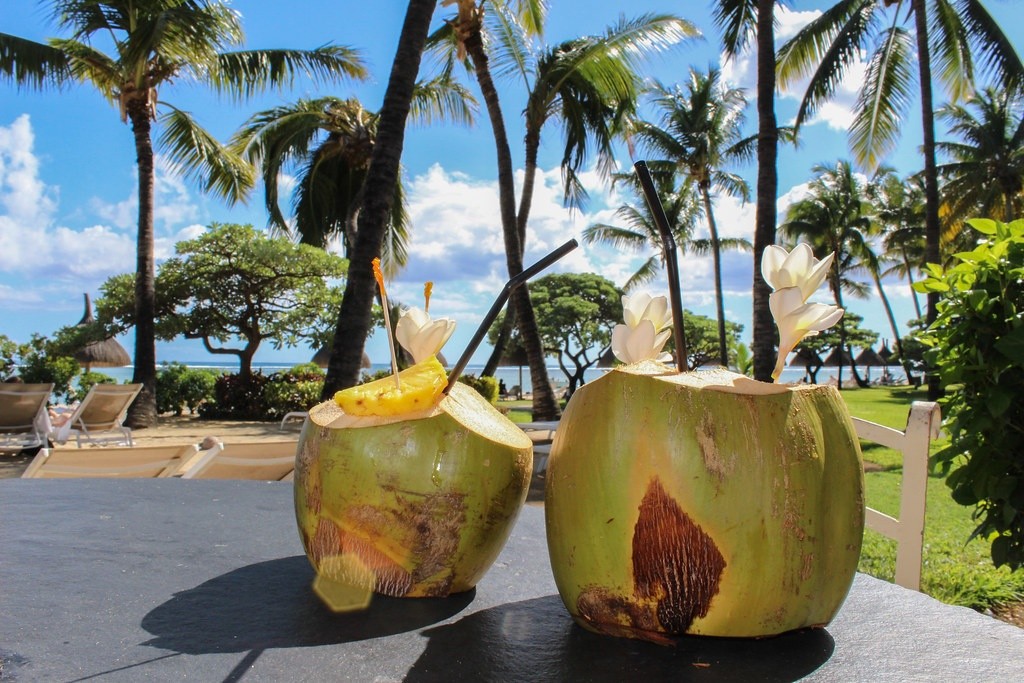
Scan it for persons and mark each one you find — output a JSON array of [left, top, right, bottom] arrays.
[[23, 388, 81, 447], [499, 379, 511, 395]]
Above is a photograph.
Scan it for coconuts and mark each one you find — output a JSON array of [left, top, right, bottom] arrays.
[[292, 375, 533, 598], [545, 360, 866, 644]]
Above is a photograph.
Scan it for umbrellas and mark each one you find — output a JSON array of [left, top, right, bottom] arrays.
[[879, 346, 902, 378], [855, 347, 887, 382], [390, 352, 448, 367], [311, 340, 371, 368], [790, 347, 824, 382], [51, 292, 131, 373], [498, 346, 531, 400], [825, 347, 854, 389]]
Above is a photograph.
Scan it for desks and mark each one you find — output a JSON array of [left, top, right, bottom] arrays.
[[2, 474, 1017, 681]]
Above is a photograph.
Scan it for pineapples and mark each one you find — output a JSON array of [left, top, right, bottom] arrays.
[[334, 353, 449, 415]]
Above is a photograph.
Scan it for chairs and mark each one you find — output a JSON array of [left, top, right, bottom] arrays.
[[56, 382, 145, 449], [0, 380, 61, 460], [851, 399, 940, 594], [499, 386, 520, 401]]
